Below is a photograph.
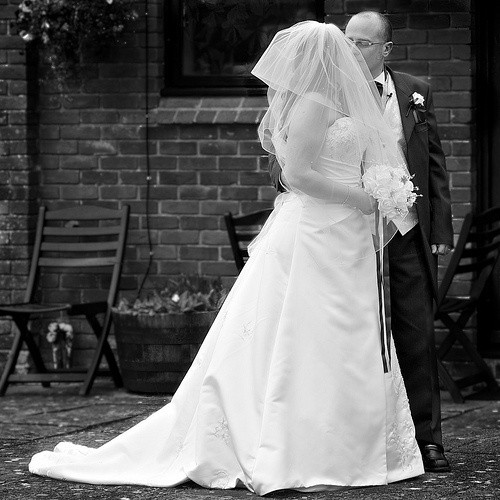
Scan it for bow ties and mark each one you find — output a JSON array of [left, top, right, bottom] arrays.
[[376, 83, 383, 96]]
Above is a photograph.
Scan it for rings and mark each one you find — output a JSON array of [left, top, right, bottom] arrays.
[[447, 249, 451, 251]]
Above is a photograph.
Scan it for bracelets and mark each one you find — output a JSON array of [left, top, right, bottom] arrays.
[[340, 185, 351, 207]]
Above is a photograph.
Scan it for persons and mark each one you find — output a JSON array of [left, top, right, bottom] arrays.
[[268, 10, 455, 474], [221, 20, 425, 496]]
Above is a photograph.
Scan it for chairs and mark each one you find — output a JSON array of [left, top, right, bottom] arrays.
[[436, 204, 500, 404], [0, 204, 131, 396], [224, 208, 273, 272]]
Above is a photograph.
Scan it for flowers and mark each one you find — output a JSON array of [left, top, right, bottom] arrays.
[[405, 92, 426, 117], [361, 165, 423, 225]]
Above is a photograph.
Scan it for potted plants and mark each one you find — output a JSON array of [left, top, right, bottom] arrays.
[[111, 275, 229, 394]]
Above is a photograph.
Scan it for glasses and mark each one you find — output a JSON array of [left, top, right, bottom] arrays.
[[352, 41, 382, 48]]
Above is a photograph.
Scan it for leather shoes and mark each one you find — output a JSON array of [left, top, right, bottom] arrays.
[[419, 444, 451, 472]]
